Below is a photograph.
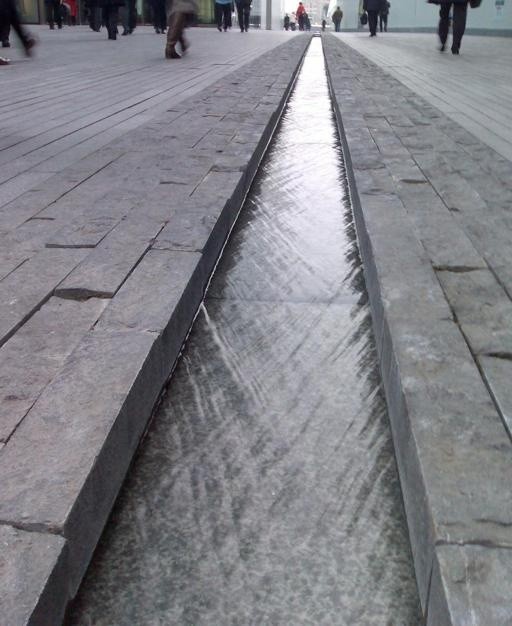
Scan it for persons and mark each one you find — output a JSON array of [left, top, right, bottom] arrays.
[[0, 0, 256, 66], [379, 0, 391, 31], [361, 0, 388, 37], [283, 0, 312, 31], [332, 5, 343, 31], [437, 0, 470, 54]]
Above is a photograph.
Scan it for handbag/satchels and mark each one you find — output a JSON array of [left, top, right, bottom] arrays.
[[359, 12, 369, 26]]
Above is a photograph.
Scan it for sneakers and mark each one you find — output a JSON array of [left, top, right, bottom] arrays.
[[0, 56, 13, 65], [165, 42, 190, 59]]
